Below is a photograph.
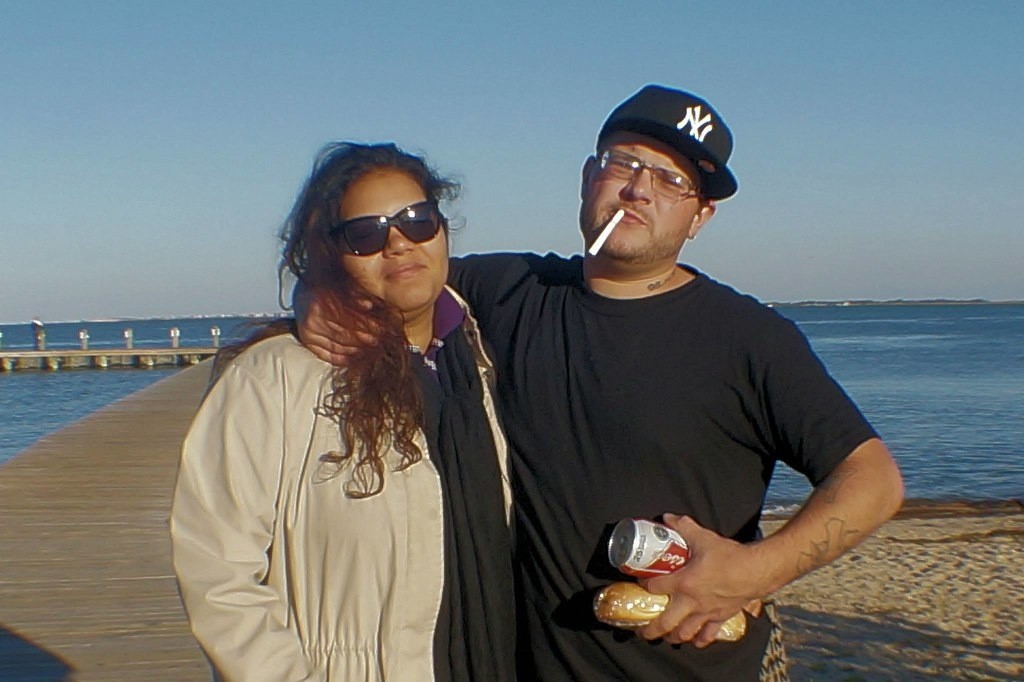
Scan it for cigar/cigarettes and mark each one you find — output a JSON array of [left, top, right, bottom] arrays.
[[588, 207, 626, 255]]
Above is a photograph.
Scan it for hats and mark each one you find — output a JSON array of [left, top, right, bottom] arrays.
[[598, 85, 737, 200]]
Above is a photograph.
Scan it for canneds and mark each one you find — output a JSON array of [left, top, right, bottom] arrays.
[[607, 517, 690, 579]]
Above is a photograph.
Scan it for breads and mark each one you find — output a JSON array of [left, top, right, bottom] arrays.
[[592, 582, 746, 641]]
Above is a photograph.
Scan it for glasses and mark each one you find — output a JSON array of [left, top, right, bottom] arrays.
[[596, 149, 702, 199], [330, 201, 440, 256]]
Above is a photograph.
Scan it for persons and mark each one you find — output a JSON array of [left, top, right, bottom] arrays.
[[168, 140, 536, 682], [294, 82, 907, 682]]
